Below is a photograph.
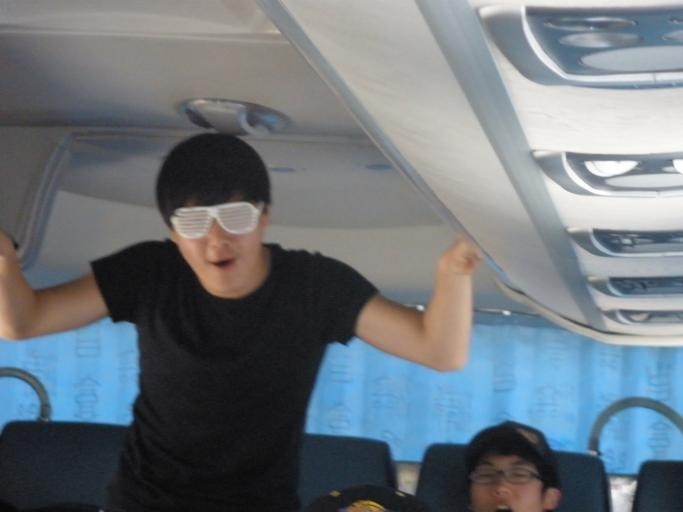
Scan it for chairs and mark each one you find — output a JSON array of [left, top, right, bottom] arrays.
[[0, 420, 683, 512]]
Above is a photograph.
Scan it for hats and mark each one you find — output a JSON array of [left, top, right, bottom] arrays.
[[464, 419, 561, 475]]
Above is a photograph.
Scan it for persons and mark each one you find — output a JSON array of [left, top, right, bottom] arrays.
[[0, 132, 479, 512], [464, 418, 563, 511]]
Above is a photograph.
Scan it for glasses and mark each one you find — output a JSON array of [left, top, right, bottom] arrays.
[[467, 466, 544, 485], [168, 200, 268, 240]]
